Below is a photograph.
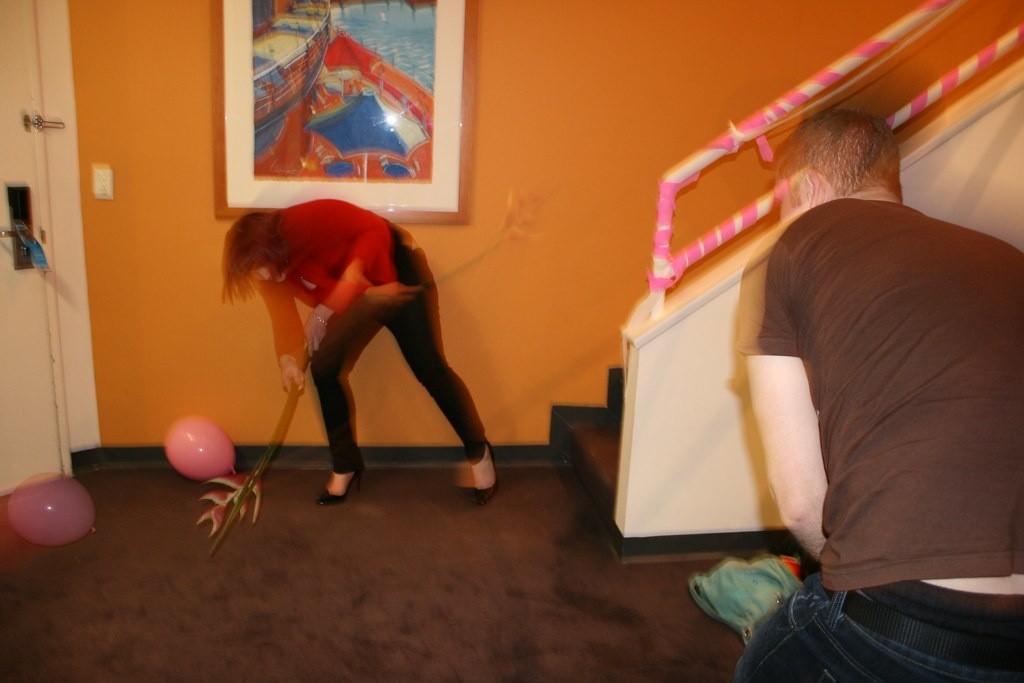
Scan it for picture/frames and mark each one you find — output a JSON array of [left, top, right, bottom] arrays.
[[210, 0, 479, 226]]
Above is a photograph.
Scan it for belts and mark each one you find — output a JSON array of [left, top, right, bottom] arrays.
[[824, 587, 1024, 673]]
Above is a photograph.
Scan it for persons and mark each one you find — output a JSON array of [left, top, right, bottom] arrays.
[[220, 199, 498, 505], [736, 106, 1024, 683]]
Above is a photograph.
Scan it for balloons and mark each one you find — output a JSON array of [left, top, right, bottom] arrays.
[[8, 472, 95, 545], [164, 415, 236, 482]]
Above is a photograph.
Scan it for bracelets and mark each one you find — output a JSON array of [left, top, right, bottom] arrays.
[[309, 313, 329, 326]]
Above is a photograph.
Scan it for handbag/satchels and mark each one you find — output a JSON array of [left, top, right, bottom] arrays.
[[688, 554, 805, 649]]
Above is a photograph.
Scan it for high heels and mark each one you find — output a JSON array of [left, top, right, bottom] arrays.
[[317, 456, 364, 505], [471, 440, 499, 505]]
